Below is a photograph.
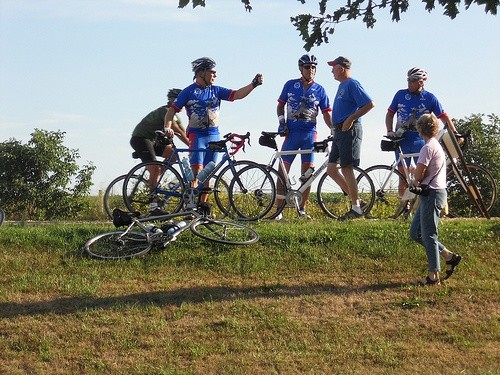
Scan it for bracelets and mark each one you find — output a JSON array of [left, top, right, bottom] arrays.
[[278, 115, 284, 120], [388, 130, 392, 132], [454, 131, 458, 134]]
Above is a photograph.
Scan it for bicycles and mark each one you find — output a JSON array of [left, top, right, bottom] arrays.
[[83, 203, 261, 260], [231, 131, 376, 221], [103, 128, 289, 225], [353, 127, 496, 221]]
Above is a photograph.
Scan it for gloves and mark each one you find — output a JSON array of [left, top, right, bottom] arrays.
[[278, 123, 288, 136]]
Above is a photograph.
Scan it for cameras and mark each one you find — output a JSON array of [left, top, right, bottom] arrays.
[[409, 183, 430, 195]]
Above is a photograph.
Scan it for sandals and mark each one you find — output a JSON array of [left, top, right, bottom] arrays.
[[444, 253, 461, 280], [412, 276, 440, 287]]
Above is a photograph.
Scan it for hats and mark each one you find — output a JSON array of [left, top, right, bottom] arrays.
[[327, 56, 351, 70]]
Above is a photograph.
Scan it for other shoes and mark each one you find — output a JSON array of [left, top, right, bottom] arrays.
[[184, 214, 196, 220], [337, 208, 364, 221], [274, 212, 283, 221], [149, 208, 169, 217], [297, 211, 313, 221], [351, 199, 368, 210], [403, 209, 412, 222]]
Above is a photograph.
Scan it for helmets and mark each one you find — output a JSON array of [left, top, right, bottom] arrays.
[[167, 88, 183, 100], [191, 57, 216, 72], [407, 66, 428, 81], [298, 54, 317, 66]]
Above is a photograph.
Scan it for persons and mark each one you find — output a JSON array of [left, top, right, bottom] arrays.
[[327, 56, 373, 219], [164, 57, 263, 219], [130, 89, 189, 217], [409, 113, 462, 284], [268, 54, 332, 218], [386, 68, 464, 220]]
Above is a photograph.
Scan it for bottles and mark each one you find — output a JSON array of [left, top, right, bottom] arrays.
[[167, 221, 186, 234], [410, 156, 417, 179], [182, 156, 194, 181], [196, 161, 215, 181], [146, 223, 163, 234], [298, 166, 315, 182]]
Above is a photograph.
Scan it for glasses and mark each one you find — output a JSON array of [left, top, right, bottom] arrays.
[[302, 66, 316, 69], [407, 78, 419, 82]]
